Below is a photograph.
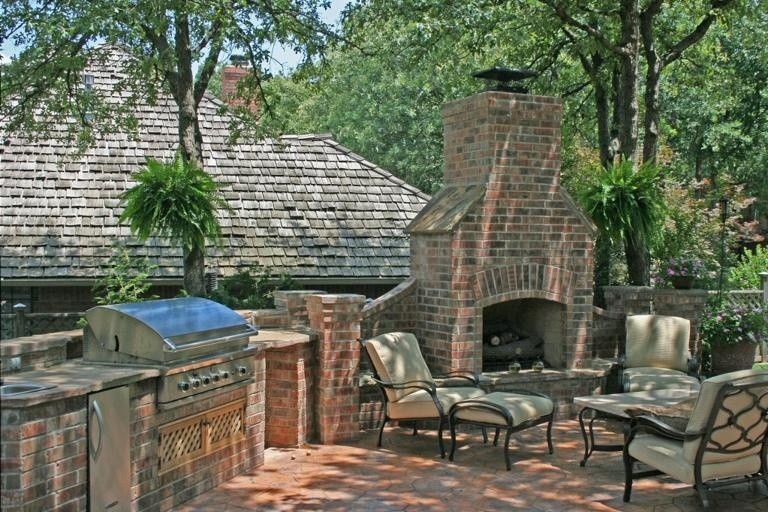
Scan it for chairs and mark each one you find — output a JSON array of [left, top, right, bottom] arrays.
[[359, 330, 486, 460], [620, 314, 701, 392], [623, 370, 767, 512]]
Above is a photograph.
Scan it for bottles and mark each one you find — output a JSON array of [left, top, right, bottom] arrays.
[[531, 355, 544, 371], [508, 360, 521, 373]]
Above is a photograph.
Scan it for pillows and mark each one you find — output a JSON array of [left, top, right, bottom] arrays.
[[623, 395, 701, 434]]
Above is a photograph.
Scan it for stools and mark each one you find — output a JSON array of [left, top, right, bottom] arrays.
[[448, 388, 556, 471]]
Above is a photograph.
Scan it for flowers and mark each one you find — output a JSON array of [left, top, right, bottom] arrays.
[[703, 303, 767, 346], [666, 259, 702, 276]]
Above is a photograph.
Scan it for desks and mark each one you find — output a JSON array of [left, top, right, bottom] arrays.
[[573, 389, 700, 469]]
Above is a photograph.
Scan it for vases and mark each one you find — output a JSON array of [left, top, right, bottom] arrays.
[[708, 345, 757, 374], [672, 276, 696, 291]]
